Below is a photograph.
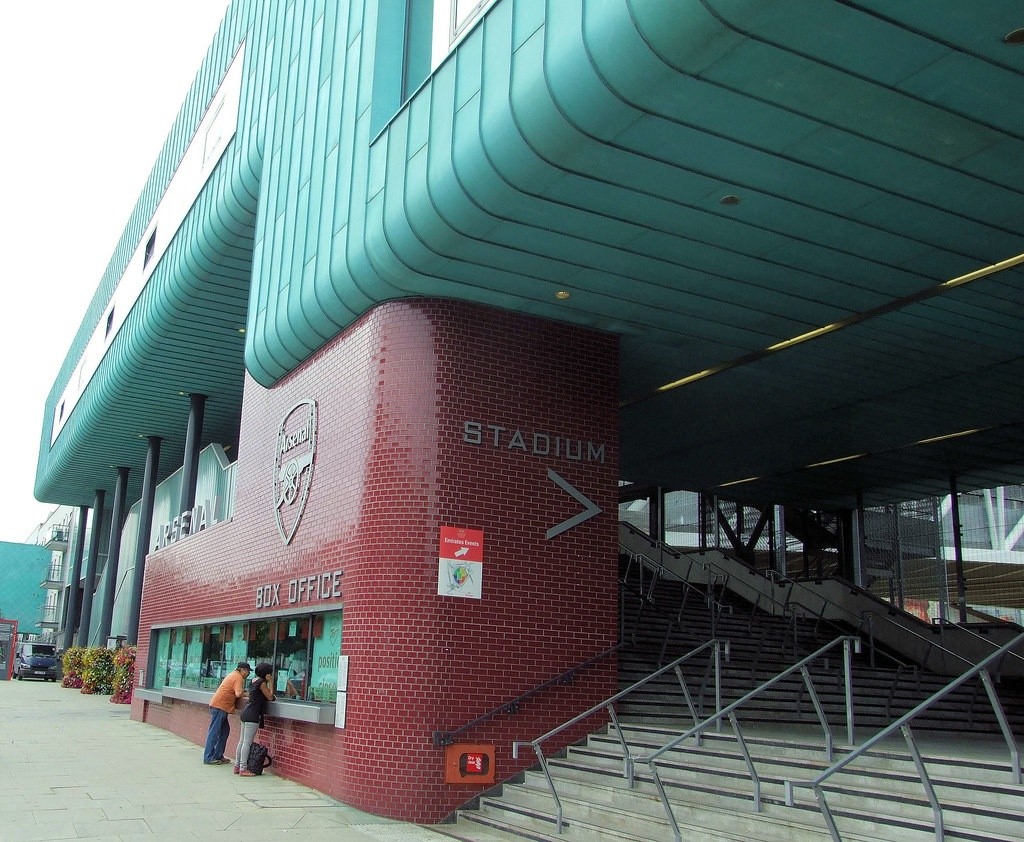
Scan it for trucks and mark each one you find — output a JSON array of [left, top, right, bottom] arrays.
[[12, 641, 58, 682]]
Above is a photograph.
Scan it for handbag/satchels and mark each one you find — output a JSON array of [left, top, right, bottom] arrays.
[[247, 741, 272, 774]]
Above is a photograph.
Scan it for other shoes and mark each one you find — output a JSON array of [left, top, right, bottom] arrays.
[[208, 758, 230, 764], [233, 766, 256, 776]]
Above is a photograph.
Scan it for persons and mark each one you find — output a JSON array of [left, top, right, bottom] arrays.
[[204, 662, 254, 766], [276, 667, 298, 697], [233, 663, 275, 776]]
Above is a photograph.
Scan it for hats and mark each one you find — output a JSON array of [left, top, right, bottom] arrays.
[[237, 662, 254, 671]]
